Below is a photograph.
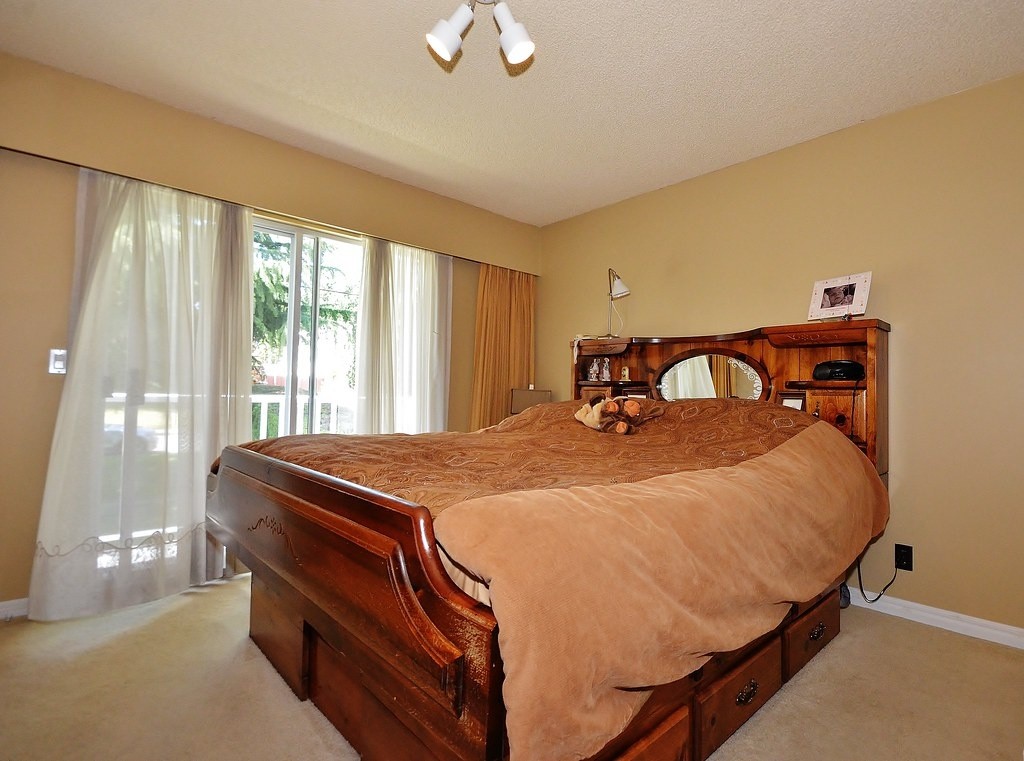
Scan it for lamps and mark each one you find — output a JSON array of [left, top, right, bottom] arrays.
[[425, 0, 476, 61], [511, 382, 553, 416], [492, 0, 535, 65], [596, 268, 630, 340]]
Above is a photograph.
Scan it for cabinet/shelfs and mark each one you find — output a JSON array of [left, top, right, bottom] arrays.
[[571, 317, 892, 494]]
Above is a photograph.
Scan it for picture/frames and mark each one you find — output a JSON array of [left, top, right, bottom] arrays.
[[808, 271, 872, 320], [620, 386, 651, 401], [775, 389, 806, 412]]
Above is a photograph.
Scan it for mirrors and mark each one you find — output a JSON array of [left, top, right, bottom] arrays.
[[649, 345, 772, 402]]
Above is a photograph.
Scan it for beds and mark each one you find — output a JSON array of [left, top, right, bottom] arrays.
[[204, 394, 926, 760]]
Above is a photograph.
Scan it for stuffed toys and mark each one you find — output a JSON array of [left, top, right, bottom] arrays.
[[574, 393, 664, 435]]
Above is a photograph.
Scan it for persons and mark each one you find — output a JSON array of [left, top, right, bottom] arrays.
[[827, 288, 853, 307]]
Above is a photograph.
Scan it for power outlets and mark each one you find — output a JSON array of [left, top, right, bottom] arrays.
[[895, 543, 913, 572]]
